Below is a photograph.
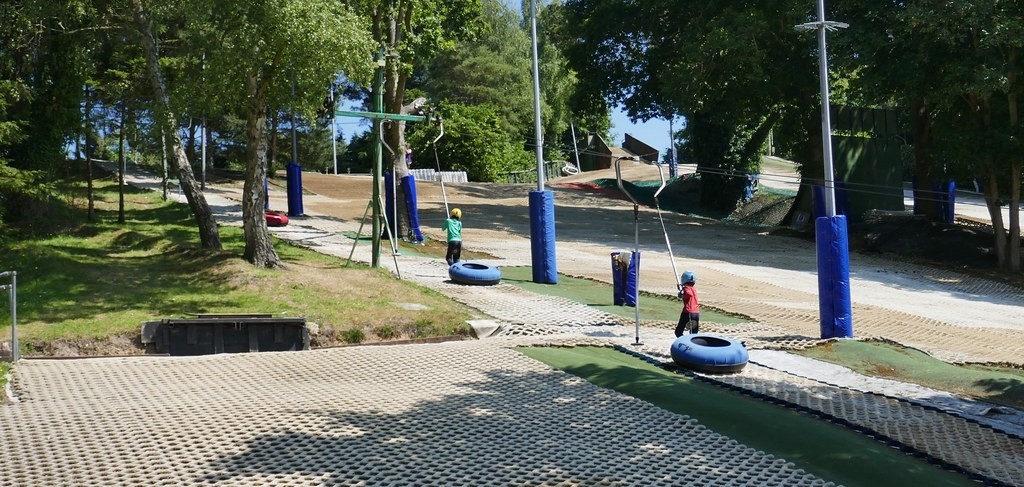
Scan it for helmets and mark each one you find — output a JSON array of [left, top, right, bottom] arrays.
[[450, 208, 462, 220], [681, 272, 695, 287]]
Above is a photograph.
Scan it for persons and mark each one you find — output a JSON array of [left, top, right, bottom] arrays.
[[674, 272, 699, 339], [442, 209, 462, 267]]
